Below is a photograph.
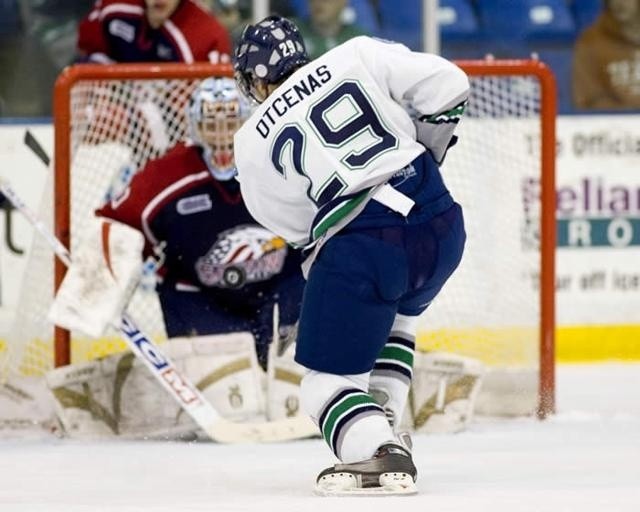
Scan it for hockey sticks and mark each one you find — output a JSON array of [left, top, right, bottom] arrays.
[[0, 178, 322, 446]]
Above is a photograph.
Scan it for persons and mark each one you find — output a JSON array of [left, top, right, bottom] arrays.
[[95, 75, 307, 373], [232, 16, 473, 489], [72, 0, 231, 145], [573, 0, 640, 109]]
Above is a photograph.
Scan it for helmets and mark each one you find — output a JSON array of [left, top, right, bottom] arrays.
[[236, 15, 308, 83], [191, 76, 251, 123]]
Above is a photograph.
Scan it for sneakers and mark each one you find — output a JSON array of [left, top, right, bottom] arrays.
[[314, 443, 418, 488]]
